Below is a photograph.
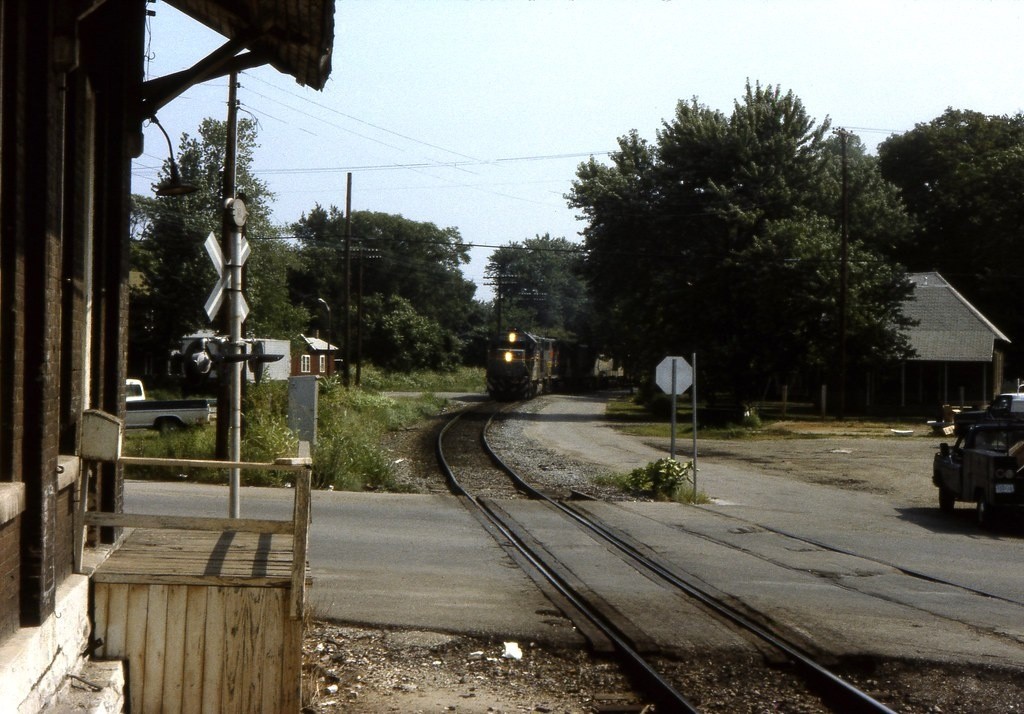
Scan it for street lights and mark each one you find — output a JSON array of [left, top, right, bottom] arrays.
[[318, 298, 331, 378]]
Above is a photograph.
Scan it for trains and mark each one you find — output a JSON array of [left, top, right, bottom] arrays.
[[483, 328, 639, 401]]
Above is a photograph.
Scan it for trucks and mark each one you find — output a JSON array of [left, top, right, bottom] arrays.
[[932, 393, 1023, 526], [125, 399, 211, 438]]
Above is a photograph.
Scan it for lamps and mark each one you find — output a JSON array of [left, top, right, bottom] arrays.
[[148, 115, 199, 196]]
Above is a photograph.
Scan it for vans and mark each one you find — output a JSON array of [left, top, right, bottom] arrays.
[[124, 379, 145, 402]]
